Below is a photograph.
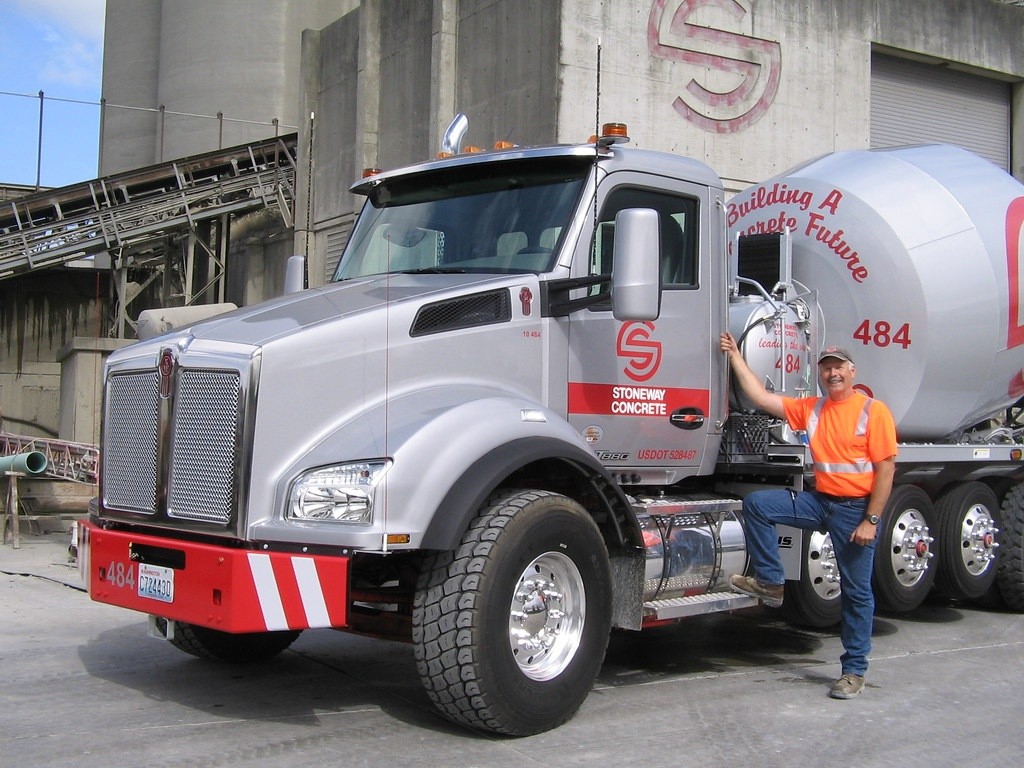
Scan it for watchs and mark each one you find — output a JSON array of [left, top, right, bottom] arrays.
[[865, 514, 881, 525]]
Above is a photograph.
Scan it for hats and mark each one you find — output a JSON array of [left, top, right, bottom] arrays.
[[817, 348, 856, 365]]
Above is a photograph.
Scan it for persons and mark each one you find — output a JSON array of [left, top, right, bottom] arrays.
[[720, 330, 899, 700]]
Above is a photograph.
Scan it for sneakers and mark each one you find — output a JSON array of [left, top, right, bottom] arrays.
[[728, 575, 784, 608], [831, 673, 865, 698]]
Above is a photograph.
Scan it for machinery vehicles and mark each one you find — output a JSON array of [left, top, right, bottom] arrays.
[[77, 36, 1024, 739]]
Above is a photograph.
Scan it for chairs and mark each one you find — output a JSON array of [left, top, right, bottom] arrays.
[[658, 215, 687, 283]]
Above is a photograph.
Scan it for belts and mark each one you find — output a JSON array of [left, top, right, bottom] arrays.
[[828, 494, 868, 503]]
